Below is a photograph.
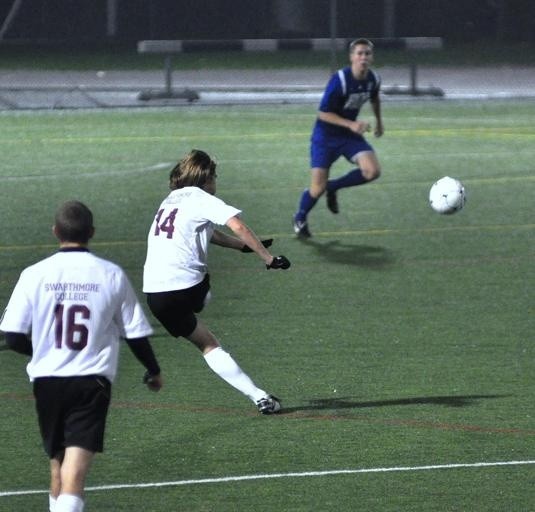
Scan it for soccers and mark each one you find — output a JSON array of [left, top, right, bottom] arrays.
[[429, 177, 467, 214]]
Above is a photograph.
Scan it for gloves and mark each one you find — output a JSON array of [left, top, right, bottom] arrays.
[[241, 239, 272, 254], [266, 256, 290, 271]]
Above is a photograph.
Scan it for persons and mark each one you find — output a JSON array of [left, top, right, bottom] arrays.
[[141, 149, 292, 417], [290, 37, 386, 242], [0, 198, 164, 512]]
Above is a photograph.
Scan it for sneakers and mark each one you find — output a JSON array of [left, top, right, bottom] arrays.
[[291, 218, 310, 238], [324, 181, 339, 214], [257, 396, 280, 414]]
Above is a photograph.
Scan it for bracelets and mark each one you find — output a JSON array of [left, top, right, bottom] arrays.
[[144, 365, 160, 380]]
